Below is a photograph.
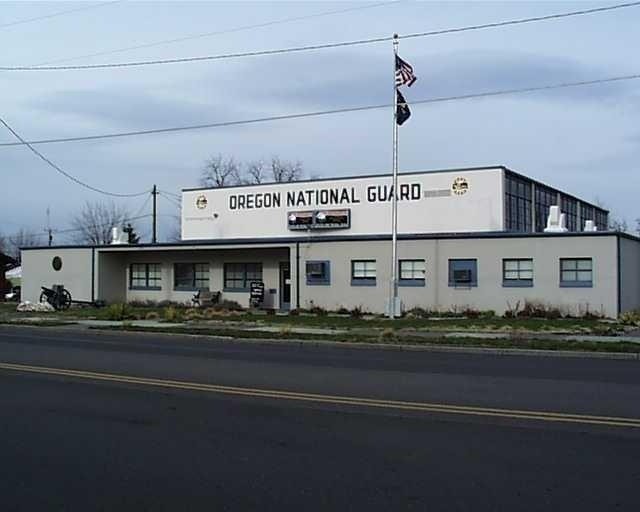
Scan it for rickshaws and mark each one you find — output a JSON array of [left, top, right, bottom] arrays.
[[39, 284, 106, 312]]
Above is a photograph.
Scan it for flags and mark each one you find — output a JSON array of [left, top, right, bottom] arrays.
[[394, 53, 415, 89], [396, 88, 411, 126]]
